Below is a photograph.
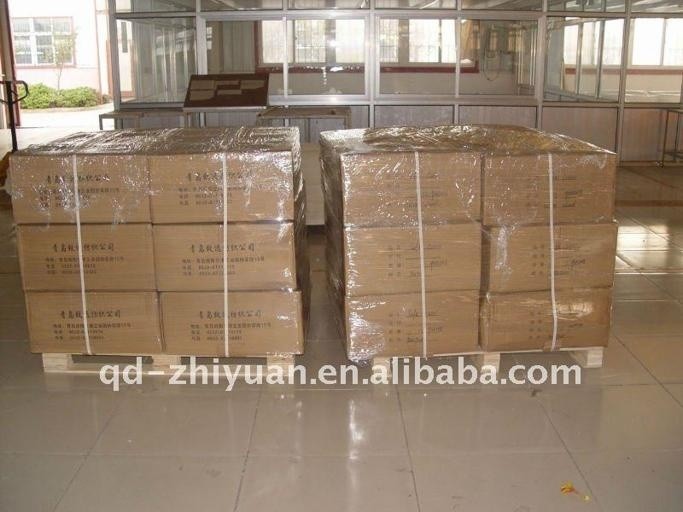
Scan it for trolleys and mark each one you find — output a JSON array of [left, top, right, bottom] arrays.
[[0, 80, 45, 205]]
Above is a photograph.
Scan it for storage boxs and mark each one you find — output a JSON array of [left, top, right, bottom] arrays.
[[7, 129, 617, 383]]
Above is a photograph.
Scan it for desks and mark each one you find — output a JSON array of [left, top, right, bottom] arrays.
[[98, 108, 193, 131], [659, 107, 682, 168], [256, 107, 351, 140]]
